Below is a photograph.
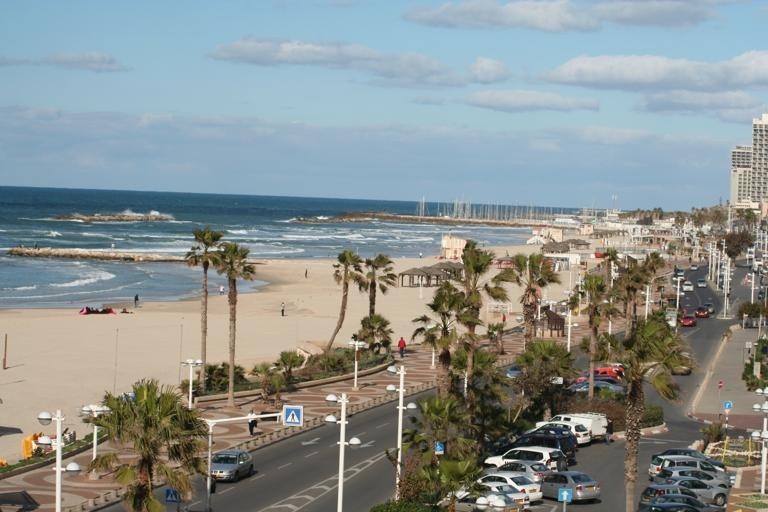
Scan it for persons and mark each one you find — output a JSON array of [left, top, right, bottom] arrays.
[[280, 301, 286, 317], [219, 284, 225, 295], [398, 336, 406, 359], [134, 293, 139, 308], [502, 312, 506, 325], [247, 408, 259, 436]]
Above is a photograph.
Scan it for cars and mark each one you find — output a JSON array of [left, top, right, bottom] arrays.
[[202, 448, 253, 482], [672, 264, 715, 328]]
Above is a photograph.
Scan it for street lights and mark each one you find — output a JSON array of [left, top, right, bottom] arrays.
[[751, 386, 768, 495], [180, 358, 203, 410], [324, 391, 361, 512], [348, 341, 366, 392], [752, 341, 760, 362], [37, 409, 81, 512], [707, 220, 768, 319], [80, 404, 111, 461], [384, 365, 418, 505], [516, 265, 655, 355]]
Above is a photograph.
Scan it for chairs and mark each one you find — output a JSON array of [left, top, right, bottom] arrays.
[[544, 309, 565, 337]]
